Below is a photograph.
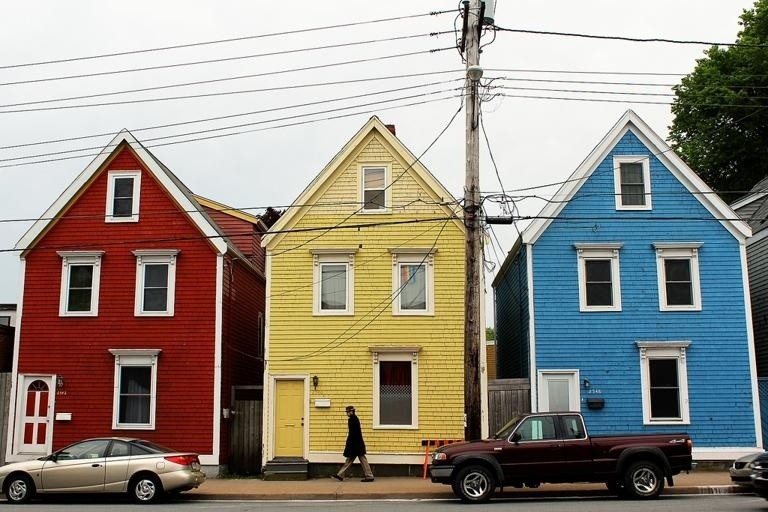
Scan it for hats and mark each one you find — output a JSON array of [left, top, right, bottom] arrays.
[[345, 406, 355, 411]]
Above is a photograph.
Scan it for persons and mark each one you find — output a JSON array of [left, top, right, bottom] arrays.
[[329, 406, 374, 482]]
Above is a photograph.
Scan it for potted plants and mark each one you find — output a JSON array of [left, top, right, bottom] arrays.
[[486, 325, 497, 346]]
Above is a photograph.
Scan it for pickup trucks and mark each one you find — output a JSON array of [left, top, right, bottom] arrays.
[[427, 411, 695, 499]]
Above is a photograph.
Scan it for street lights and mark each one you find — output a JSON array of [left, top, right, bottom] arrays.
[[460, 60, 487, 440]]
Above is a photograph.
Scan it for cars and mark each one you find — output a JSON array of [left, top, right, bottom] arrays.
[[727, 451, 767, 486], [747, 454, 768, 500], [0, 436, 204, 504]]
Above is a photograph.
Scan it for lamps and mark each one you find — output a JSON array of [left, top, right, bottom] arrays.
[[57, 372, 64, 388], [312, 374, 319, 391]]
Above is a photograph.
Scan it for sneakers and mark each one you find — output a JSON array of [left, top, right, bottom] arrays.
[[362, 479, 374, 481], [331, 474, 343, 481]]
